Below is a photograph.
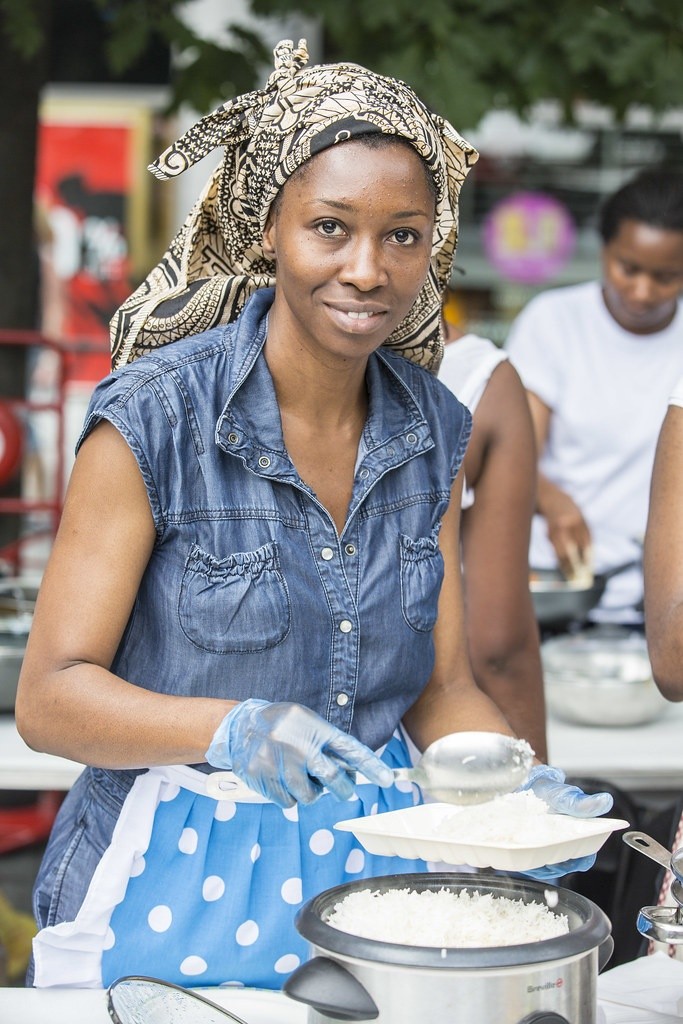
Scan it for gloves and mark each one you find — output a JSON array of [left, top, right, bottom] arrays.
[[204, 697, 393, 808], [509, 766, 613, 877]]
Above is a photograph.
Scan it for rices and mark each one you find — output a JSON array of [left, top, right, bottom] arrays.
[[452, 737, 552, 845], [325, 886, 569, 959]]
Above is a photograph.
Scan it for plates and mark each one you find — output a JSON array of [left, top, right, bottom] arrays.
[[334, 802, 632, 873]]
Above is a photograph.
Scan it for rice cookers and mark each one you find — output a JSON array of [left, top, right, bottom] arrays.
[[284, 868, 615, 1024]]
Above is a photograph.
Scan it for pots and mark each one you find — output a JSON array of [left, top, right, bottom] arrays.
[[621, 829, 683, 971], [531, 552, 640, 628]]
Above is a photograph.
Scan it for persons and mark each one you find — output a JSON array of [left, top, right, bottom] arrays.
[[430, 320, 549, 765], [502, 160, 683, 626], [644, 370, 683, 700], [15, 38, 615, 997]]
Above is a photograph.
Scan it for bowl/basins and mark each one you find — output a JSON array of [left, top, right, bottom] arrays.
[[1, 628, 36, 717], [540, 636, 670, 730]]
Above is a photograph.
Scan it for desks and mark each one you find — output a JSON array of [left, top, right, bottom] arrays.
[[0, 714, 86, 792], [543, 635, 683, 791]]
